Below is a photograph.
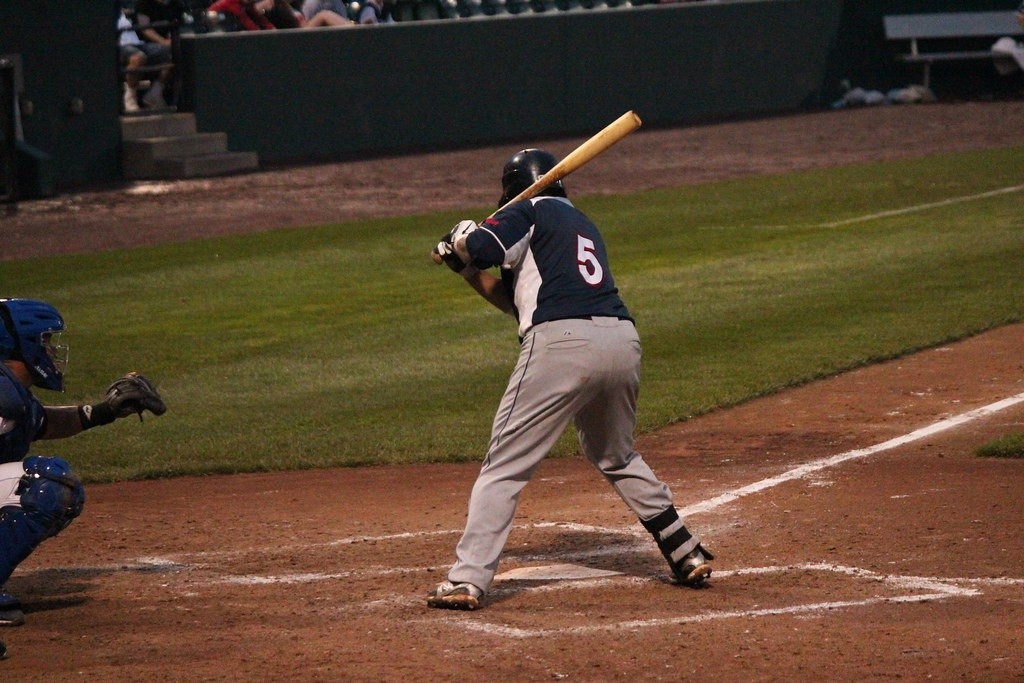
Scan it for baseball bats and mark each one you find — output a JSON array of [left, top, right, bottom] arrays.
[[432, 109, 644, 268]]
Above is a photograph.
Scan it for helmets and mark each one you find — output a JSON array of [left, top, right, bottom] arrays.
[[0, 297, 70, 394], [497, 148, 566, 208]]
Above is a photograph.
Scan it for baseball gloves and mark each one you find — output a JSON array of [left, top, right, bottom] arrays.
[[102, 369, 167, 423]]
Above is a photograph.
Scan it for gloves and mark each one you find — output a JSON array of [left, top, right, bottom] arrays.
[[437, 220, 479, 264]]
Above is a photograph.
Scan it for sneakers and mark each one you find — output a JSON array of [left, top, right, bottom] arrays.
[[0, 584, 25, 626], [427, 582, 483, 611], [668, 550, 712, 585]]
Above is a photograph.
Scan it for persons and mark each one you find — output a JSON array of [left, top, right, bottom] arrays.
[[116, 0, 398, 120], [0, 296, 166, 628], [425, 148, 715, 607]]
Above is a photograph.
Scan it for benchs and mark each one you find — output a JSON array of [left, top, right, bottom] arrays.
[[884, 11, 1024, 89]]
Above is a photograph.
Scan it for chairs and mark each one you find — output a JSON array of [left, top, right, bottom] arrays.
[[119, 0, 652, 73]]
